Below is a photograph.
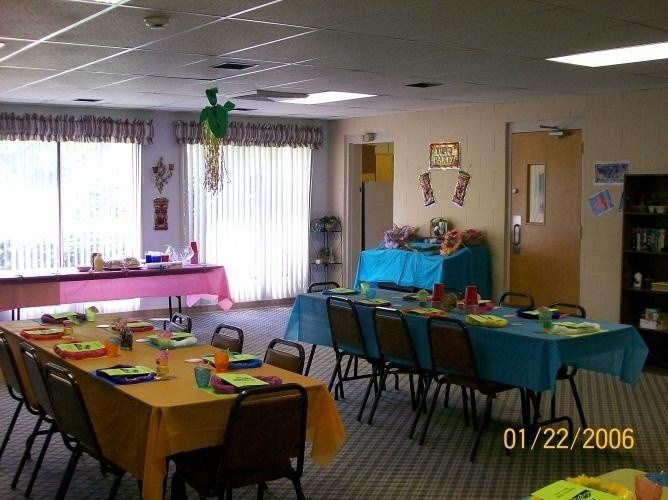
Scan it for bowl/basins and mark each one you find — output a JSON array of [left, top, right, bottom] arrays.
[[77, 267, 91, 272]]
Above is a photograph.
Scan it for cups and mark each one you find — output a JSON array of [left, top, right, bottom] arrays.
[[91, 252, 101, 271], [145, 254, 170, 263], [431, 283, 444, 308], [543, 321, 553, 333], [214, 351, 230, 372], [85, 308, 97, 322], [104, 338, 119, 356], [361, 282, 376, 299], [463, 285, 479, 317], [194, 366, 211, 388]]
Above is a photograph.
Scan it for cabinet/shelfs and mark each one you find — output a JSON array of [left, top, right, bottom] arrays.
[[620, 174, 668, 369]]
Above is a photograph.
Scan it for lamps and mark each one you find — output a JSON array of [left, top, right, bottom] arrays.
[[151, 157, 175, 194]]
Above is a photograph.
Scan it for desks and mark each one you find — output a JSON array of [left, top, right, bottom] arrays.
[[353, 238, 493, 299], [282, 287, 650, 442], [0, 313, 347, 498], [596, 468, 668, 500], [0, 262, 234, 325]]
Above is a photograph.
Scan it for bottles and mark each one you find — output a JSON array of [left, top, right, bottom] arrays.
[[63, 320, 74, 339], [155, 357, 168, 379], [190, 242, 199, 264], [95, 254, 104, 271]]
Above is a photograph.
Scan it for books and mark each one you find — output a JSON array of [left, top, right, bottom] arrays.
[[633, 228, 665, 252]]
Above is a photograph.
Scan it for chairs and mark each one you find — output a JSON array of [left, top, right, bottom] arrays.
[[408, 315, 541, 463], [356, 307, 469, 426], [210, 323, 244, 353], [10, 343, 116, 500], [167, 312, 191, 334], [0, 330, 47, 461], [262, 338, 305, 375], [325, 296, 399, 399], [498, 292, 535, 311], [24, 361, 143, 500], [305, 281, 340, 377], [161, 383, 308, 499], [527, 302, 587, 432]]
